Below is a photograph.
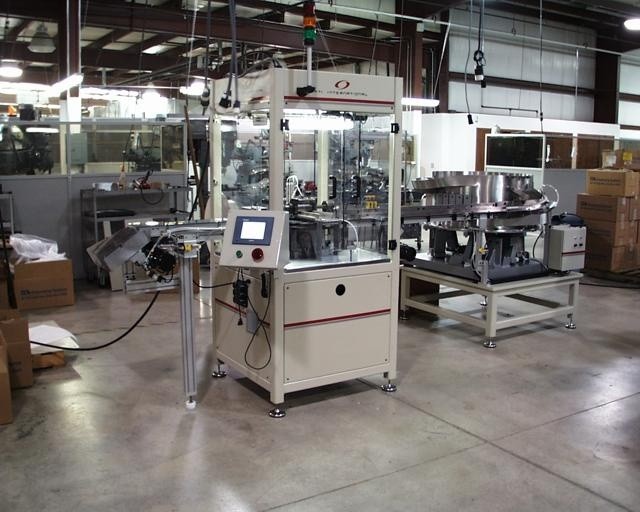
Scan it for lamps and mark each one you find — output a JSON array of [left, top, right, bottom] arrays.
[[1, 18, 56, 80]]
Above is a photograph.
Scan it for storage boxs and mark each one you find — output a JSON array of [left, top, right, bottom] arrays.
[[573, 148, 640, 273], [1, 248, 76, 425]]
